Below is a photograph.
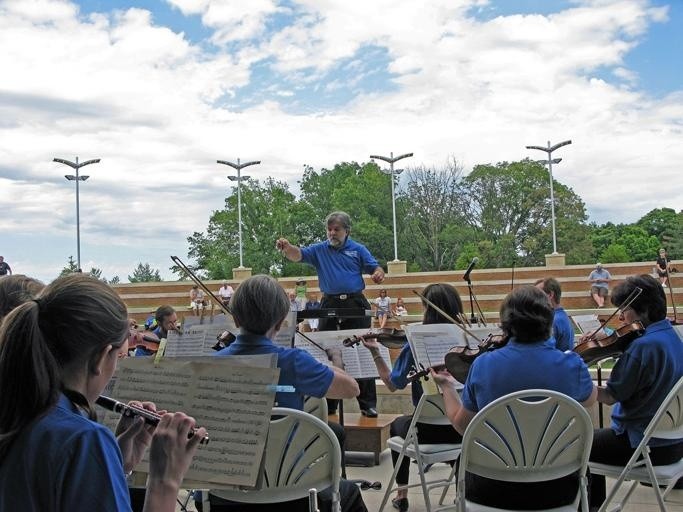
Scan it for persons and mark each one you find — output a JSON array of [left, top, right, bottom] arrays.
[[586, 262, 612, 309], [655, 249, 669, 288], [286, 277, 322, 332], [218, 281, 233, 313], [0, 272, 207, 512], [207, 275, 369, 511], [533, 277, 574, 353], [0, 256, 11, 276], [392, 298, 405, 316], [575, 273, 683, 511], [189, 285, 203, 317], [429, 285, 594, 511], [358, 283, 470, 512], [118, 305, 180, 358], [274, 210, 386, 417], [375, 288, 391, 329], [599, 319, 613, 336]]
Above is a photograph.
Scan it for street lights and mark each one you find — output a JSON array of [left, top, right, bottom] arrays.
[[215, 157, 261, 271], [524, 138, 575, 257], [53, 155, 102, 272], [367, 152, 415, 265]]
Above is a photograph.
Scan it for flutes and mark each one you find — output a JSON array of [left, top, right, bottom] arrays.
[[94, 395, 209, 445]]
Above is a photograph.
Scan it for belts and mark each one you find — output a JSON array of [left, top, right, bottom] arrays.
[[322, 291, 365, 300]]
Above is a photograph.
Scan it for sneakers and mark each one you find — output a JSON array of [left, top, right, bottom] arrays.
[[662, 283, 667, 287], [599, 305, 604, 309]]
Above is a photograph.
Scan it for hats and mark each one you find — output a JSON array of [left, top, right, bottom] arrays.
[[296, 277, 307, 286]]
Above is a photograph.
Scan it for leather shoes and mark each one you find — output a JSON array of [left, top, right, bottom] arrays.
[[392, 496, 409, 511], [327, 405, 336, 415], [361, 407, 378, 418]]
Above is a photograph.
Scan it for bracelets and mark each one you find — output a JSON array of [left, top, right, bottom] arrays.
[[373, 354, 382, 361]]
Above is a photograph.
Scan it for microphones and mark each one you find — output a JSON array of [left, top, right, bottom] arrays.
[[462, 256, 480, 280]]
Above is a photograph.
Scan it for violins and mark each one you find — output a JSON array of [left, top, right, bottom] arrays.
[[128, 328, 160, 352], [342, 327, 407, 350], [571, 318, 646, 368], [405, 333, 508, 385]]
